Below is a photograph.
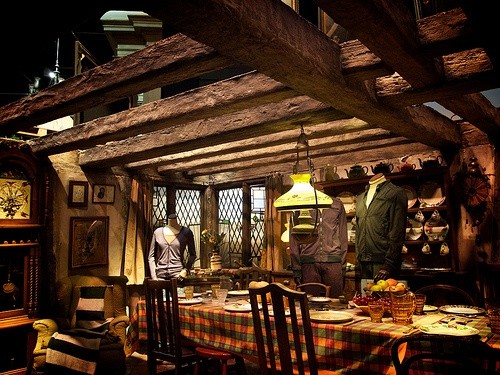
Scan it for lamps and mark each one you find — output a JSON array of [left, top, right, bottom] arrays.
[[273, 120, 334, 245]]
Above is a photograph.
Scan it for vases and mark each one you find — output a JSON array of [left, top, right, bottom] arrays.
[[208, 248, 222, 271]]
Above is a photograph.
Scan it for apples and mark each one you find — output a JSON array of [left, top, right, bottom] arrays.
[[367, 278, 405, 291]]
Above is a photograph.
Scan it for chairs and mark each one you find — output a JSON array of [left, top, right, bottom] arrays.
[[142, 266, 497, 375]]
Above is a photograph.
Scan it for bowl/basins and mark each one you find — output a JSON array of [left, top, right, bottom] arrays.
[[363, 287, 410, 300]]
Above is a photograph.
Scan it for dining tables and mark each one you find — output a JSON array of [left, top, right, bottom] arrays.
[[124, 298, 500, 375]]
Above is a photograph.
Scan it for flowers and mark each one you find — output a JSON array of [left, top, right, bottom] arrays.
[[201, 229, 226, 249]]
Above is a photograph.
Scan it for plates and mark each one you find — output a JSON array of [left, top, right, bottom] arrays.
[[336, 192, 355, 213], [268, 310, 290, 317], [309, 297, 331, 302], [419, 324, 479, 336], [178, 299, 203, 305], [401, 185, 417, 209], [178, 293, 201, 298], [227, 290, 249, 295], [224, 304, 262, 312], [418, 181, 446, 207], [310, 311, 353, 323], [423, 305, 438, 311], [439, 305, 485, 314]]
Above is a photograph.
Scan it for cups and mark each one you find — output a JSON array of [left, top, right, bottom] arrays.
[[422, 243, 430, 253], [414, 294, 426, 314], [211, 285, 220, 300], [185, 286, 194, 301], [440, 242, 449, 256], [390, 293, 416, 325], [402, 244, 408, 253], [216, 289, 228, 305], [369, 301, 384, 323]]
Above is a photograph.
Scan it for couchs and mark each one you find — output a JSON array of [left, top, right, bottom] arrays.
[[32, 276, 130, 375]]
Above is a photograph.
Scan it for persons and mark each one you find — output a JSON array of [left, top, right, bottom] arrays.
[[148, 214, 196, 298], [289, 194, 348, 298], [355, 173, 408, 281]]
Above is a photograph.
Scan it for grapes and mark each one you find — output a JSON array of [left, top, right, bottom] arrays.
[[353, 293, 393, 307]]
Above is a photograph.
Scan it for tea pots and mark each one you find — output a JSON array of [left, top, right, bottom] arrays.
[[370, 162, 394, 175], [319, 163, 339, 182], [396, 154, 443, 172], [344, 164, 368, 178]]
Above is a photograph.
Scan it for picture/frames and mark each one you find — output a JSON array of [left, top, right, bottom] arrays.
[[68, 180, 89, 208], [92, 184, 116, 205], [68, 216, 109, 268]]
